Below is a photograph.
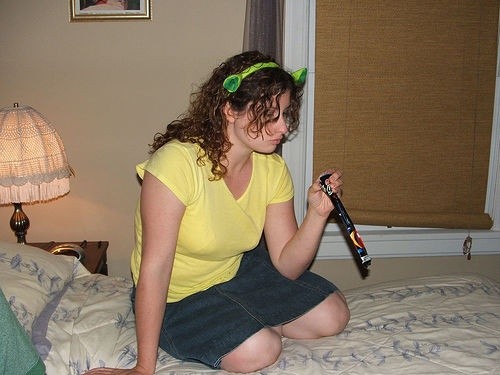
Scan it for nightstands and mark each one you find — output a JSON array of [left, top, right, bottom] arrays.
[[21, 241, 109, 275]]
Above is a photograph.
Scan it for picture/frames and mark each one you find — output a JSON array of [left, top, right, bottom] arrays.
[[68, 0, 152, 23]]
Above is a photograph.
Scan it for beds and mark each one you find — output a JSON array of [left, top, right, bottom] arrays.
[[0, 241, 500, 375]]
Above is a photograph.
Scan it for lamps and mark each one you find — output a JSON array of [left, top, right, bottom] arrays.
[[0, 104, 75, 244]]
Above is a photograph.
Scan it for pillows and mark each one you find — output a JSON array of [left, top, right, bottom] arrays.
[[0, 244, 73, 363]]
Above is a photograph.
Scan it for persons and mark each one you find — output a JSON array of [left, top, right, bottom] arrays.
[[83, 50, 351, 375]]
